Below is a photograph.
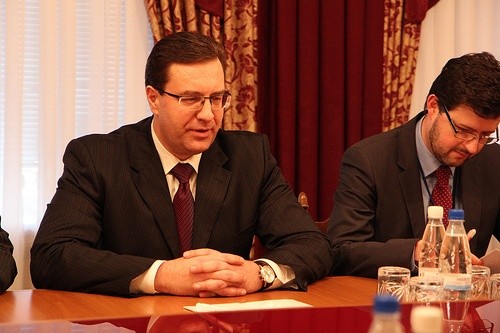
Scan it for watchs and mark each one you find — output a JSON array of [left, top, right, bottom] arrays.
[[254, 261, 276, 292]]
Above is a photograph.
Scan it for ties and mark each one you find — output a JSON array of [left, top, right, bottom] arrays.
[[170, 163, 195, 255], [429, 167, 452, 230]]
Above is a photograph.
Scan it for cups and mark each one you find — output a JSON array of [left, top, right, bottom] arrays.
[[471, 265, 500, 300], [378, 266, 440, 302], [440, 280, 469, 333], [410, 286, 444, 333]]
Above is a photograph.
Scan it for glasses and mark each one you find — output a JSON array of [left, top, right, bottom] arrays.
[[159, 88, 232, 109], [442, 104, 500, 145]]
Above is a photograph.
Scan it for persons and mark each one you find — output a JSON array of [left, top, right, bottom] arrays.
[[327, 52, 500, 279], [30, 32, 333, 298]]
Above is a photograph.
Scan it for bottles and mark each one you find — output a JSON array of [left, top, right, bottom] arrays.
[[420, 206, 471, 298], [368, 297, 405, 333]]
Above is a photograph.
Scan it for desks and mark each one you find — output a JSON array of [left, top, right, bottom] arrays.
[[0, 276, 500, 333]]
